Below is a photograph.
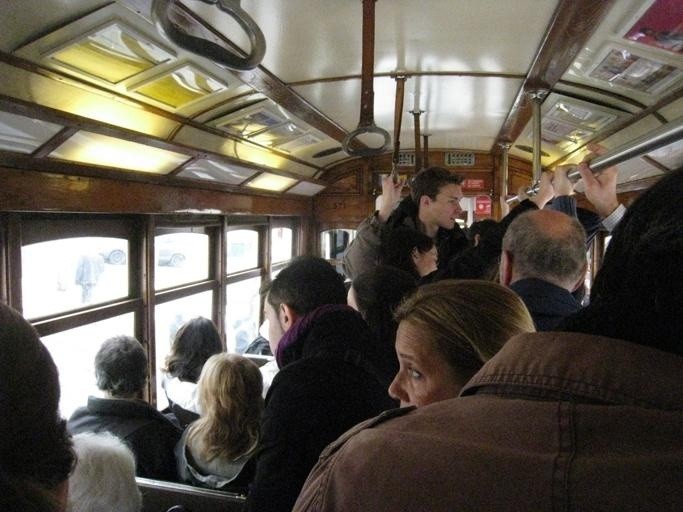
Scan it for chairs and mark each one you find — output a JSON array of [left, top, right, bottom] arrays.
[[135, 474, 251, 512]]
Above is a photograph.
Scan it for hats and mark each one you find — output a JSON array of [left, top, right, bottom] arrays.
[[0, 300, 66, 491]]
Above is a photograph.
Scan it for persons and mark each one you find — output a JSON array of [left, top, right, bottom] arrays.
[[68, 336, 174, 482], [0, 302, 78, 511], [163, 313, 223, 429], [65, 431, 143, 512], [173, 356, 264, 494], [249, 258, 387, 511], [293, 145, 681, 511]]
